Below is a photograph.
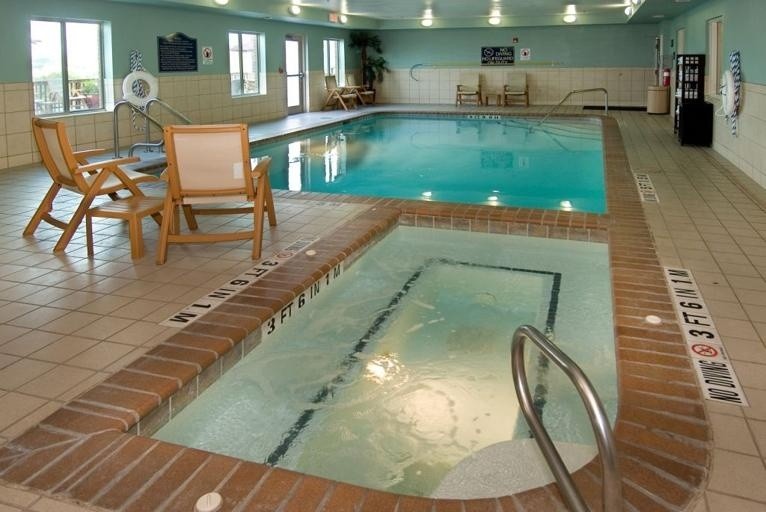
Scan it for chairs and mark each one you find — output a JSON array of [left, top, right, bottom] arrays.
[[453, 69, 483, 107], [20, 116, 163, 255], [322, 75, 358, 113], [154, 123, 278, 266], [244, 71, 257, 94], [34, 74, 100, 113], [502, 71, 530, 107], [345, 74, 375, 107]]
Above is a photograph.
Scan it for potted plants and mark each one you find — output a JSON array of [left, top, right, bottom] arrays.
[[359, 54, 390, 102]]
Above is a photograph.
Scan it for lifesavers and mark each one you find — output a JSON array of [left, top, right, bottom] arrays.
[[720, 72, 734, 117], [123, 72, 159, 108]]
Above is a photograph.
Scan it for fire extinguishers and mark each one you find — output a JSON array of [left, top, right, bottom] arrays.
[[662, 67, 670, 87]]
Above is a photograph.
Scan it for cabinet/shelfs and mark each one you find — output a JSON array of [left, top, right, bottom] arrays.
[[672, 54, 713, 146]]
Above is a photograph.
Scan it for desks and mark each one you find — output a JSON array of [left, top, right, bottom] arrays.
[[484, 93, 502, 107], [85, 195, 180, 261]]
